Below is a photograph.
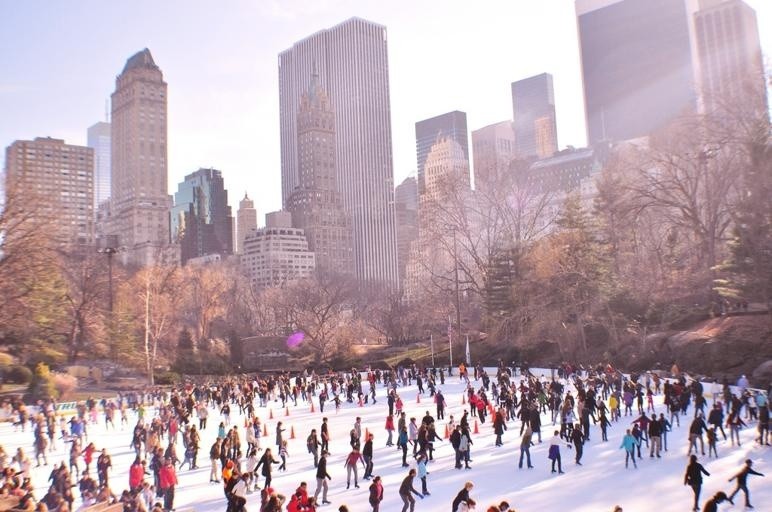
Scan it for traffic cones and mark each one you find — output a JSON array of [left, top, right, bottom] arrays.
[[364, 427, 369, 443], [284, 405, 289, 416], [461, 394, 466, 404], [443, 424, 449, 439], [251, 412, 256, 423], [310, 403, 315, 414], [416, 393, 422, 404], [269, 408, 273, 420], [243, 415, 249, 428], [326, 430, 333, 441], [261, 423, 269, 438], [472, 420, 479, 434], [487, 399, 497, 423], [287, 425, 296, 440], [359, 396, 363, 408]]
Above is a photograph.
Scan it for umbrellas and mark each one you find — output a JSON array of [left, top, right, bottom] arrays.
[[286, 331, 305, 349]]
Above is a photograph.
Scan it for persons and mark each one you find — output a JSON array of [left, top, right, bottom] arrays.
[[1, 359, 772, 512]]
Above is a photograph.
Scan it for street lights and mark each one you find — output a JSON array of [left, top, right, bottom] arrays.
[[95, 244, 131, 361]]
